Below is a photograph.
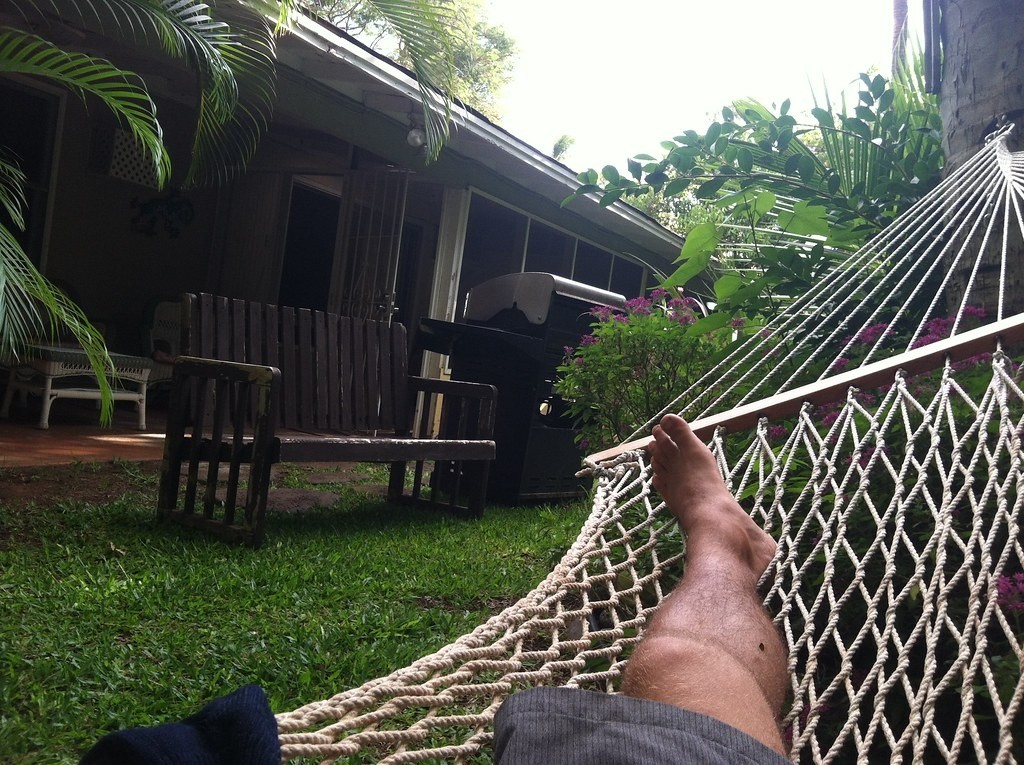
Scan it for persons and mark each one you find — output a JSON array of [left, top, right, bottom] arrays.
[[493, 414, 793, 765]]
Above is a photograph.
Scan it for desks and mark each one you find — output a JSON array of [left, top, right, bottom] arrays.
[[0, 338, 154, 430]]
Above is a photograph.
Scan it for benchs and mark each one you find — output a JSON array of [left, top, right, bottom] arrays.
[[157, 291, 497, 551]]
